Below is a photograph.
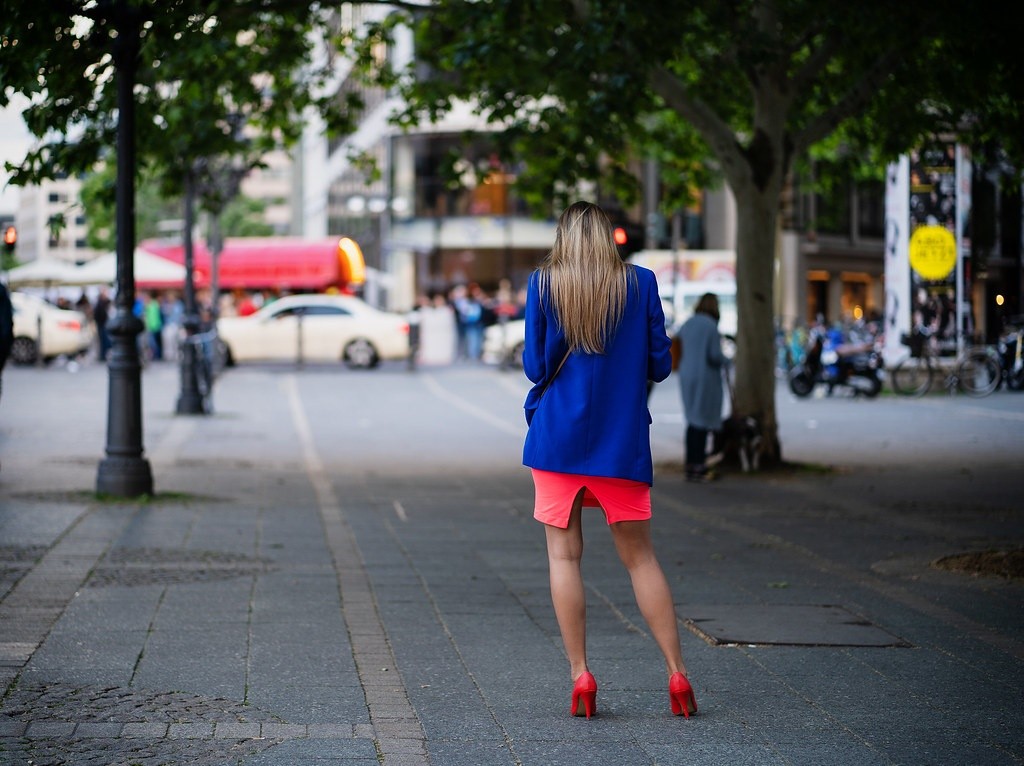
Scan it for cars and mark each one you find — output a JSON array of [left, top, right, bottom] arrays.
[[7, 291, 94, 369], [482, 318, 529, 370], [215, 292, 423, 371]]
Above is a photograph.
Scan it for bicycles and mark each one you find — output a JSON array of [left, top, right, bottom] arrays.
[[890, 323, 1003, 399]]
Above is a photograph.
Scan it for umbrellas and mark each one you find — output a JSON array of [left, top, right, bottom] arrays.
[[9, 247, 203, 283]]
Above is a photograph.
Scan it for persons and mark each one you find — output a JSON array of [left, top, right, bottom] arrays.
[[772, 316, 879, 368], [407, 305, 421, 369], [522, 202, 698, 718], [0, 284, 14, 374], [417, 278, 527, 362], [675, 293, 731, 482], [970, 328, 982, 344], [53, 287, 276, 362], [997, 325, 1024, 392]]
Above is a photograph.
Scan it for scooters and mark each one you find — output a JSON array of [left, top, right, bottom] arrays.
[[788, 329, 883, 402]]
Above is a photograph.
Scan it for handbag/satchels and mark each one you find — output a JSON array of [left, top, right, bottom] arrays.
[[668, 336, 681, 372]]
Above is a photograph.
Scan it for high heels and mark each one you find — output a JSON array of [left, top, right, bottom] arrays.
[[669, 673, 698, 720], [571, 672, 597, 721]]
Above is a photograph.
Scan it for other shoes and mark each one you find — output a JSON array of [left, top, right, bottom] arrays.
[[686, 466, 719, 482]]
